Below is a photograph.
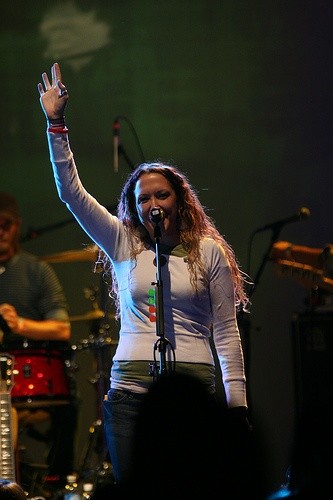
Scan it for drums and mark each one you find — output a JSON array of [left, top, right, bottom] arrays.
[[1, 344, 79, 408]]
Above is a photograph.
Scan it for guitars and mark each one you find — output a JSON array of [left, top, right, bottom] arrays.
[[0, 352, 19, 489], [275, 258, 333, 293]]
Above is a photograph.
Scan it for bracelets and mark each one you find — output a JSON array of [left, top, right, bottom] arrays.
[[48, 128, 68, 133]]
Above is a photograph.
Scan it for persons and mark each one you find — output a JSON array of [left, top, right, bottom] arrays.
[[38, 63, 248, 470], [0, 193, 71, 456], [91, 370, 333, 500]]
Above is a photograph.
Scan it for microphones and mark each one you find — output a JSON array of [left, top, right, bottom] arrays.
[[259, 207, 311, 232], [112, 120, 120, 172], [149, 206, 165, 225]]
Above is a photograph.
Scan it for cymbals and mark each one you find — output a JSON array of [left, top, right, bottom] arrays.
[[55, 309, 119, 322], [36, 244, 105, 265]]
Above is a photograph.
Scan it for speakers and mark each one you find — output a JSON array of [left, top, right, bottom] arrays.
[[292, 313, 333, 500]]
